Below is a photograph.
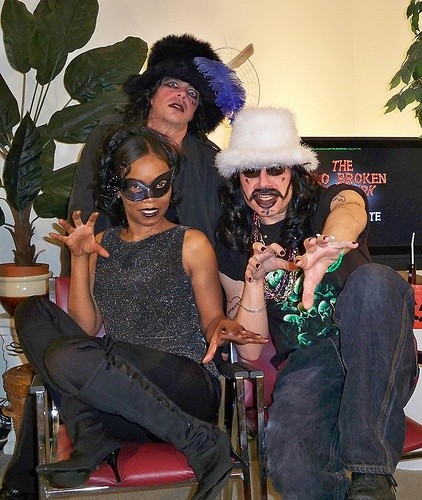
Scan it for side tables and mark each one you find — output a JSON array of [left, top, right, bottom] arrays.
[[0, 312, 28, 426]]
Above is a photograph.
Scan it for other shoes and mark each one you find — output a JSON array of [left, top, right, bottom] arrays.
[[347, 471, 398, 500]]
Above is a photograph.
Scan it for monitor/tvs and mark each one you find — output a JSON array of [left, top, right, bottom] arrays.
[[299, 136, 422, 272]]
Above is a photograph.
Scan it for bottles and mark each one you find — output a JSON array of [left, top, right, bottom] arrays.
[[408, 264, 415, 285]]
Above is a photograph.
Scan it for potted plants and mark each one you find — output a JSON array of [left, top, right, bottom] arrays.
[[0, 0, 148, 313]]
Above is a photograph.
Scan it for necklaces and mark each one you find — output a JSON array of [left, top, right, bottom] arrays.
[[250, 208, 301, 302]]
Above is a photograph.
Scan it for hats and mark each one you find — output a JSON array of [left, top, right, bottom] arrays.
[[122, 33, 254, 139], [193, 57, 320, 179]]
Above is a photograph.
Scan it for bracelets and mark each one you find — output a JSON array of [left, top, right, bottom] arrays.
[[327, 251, 343, 273], [240, 304, 266, 313]]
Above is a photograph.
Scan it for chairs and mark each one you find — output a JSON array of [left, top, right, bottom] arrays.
[[29, 277, 265, 500], [230, 337, 422, 500]]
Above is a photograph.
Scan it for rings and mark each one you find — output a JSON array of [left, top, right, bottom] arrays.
[[329, 235, 337, 246]]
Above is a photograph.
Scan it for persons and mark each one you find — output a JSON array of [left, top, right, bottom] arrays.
[[65, 31, 245, 251], [12, 126, 268, 500], [211, 106, 419, 500]]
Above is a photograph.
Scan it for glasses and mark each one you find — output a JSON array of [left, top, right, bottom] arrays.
[[242, 164, 286, 178]]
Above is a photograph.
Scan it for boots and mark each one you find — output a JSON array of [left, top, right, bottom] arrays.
[[18, 313, 121, 488], [75, 354, 251, 500]]
